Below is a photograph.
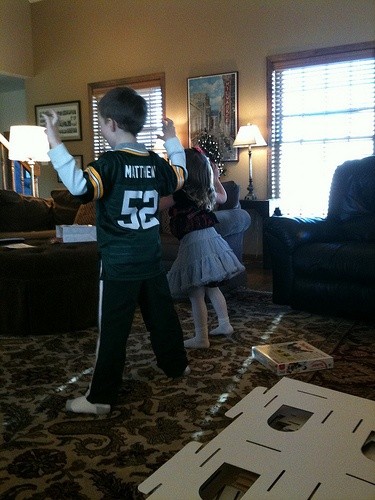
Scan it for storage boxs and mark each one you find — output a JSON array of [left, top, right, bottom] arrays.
[[251, 339, 335, 376]]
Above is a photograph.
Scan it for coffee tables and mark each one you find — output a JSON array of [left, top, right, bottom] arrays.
[[1, 239, 99, 335]]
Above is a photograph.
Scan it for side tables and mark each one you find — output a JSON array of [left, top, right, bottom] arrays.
[[239, 199, 285, 269]]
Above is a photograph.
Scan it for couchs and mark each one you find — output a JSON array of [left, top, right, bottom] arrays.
[[1, 180, 251, 294], [262, 156, 375, 324]]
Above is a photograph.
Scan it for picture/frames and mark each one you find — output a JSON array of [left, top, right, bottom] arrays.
[[34, 100, 82, 143], [57, 155, 83, 183], [187, 70, 240, 162]]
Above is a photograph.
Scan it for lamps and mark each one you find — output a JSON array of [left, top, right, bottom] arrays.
[[231, 122, 268, 201], [8, 125, 51, 198]]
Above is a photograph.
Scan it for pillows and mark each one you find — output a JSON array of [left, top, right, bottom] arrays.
[[50, 189, 83, 229], [0, 189, 54, 232]]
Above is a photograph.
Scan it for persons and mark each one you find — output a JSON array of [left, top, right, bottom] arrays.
[[149, 146, 246, 346], [40, 86, 191, 413]]
